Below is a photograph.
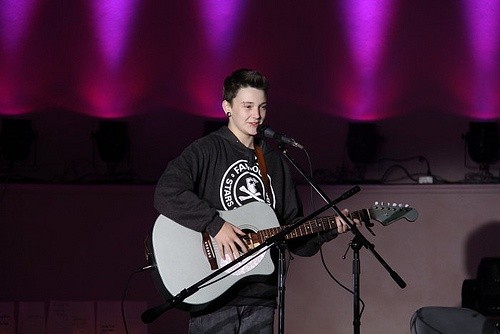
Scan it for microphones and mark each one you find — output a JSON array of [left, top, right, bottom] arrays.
[[257, 124, 304, 149], [141, 287, 200, 324]]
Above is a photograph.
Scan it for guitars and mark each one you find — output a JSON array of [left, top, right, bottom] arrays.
[[147, 202, 418, 314]]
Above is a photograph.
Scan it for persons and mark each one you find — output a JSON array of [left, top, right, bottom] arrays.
[[153, 69, 361, 334]]
